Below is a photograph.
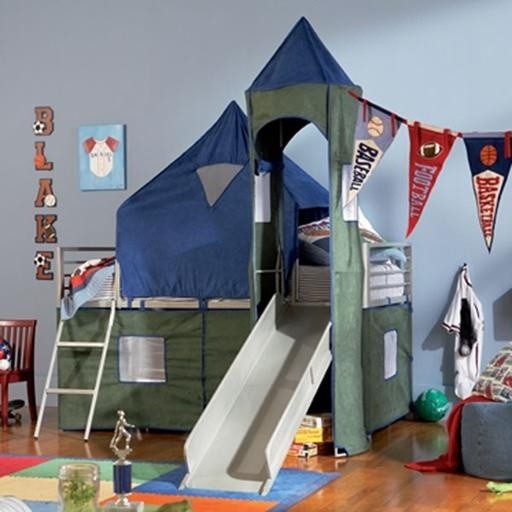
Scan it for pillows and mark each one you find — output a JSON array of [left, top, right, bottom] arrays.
[[472, 346, 512, 400]]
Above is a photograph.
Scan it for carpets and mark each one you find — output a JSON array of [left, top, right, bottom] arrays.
[[0, 451, 345, 512]]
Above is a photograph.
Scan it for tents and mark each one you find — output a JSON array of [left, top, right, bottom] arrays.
[[58, 99, 412, 456]]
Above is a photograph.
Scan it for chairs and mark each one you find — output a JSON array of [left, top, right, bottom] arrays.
[[0, 315, 39, 430]]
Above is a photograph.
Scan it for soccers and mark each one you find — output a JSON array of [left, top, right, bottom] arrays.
[[415, 387, 448, 422]]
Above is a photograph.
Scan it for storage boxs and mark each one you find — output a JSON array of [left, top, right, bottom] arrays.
[[292, 412, 333, 444]]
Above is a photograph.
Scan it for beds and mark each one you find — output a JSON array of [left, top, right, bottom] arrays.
[[57, 243, 415, 435]]
